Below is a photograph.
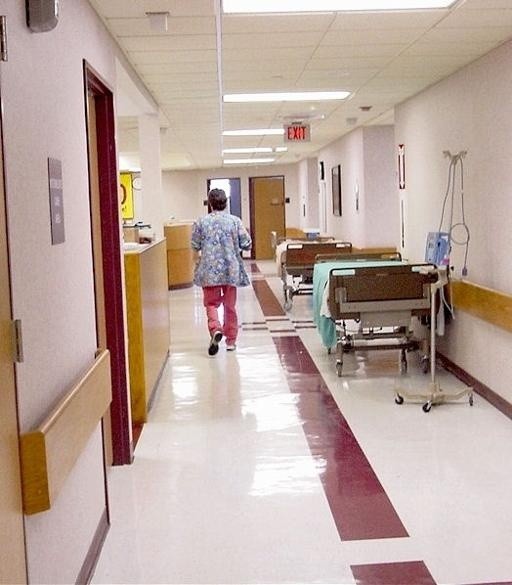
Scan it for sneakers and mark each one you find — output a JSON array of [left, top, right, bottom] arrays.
[[208, 331, 236, 355]]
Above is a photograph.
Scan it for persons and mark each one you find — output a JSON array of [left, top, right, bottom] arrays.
[[192, 188, 253, 355]]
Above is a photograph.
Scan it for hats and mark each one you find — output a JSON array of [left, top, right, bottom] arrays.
[[208, 188, 227, 200]]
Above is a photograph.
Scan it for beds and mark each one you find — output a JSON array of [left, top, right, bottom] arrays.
[[312, 252, 438, 378], [269, 228, 351, 309]]
